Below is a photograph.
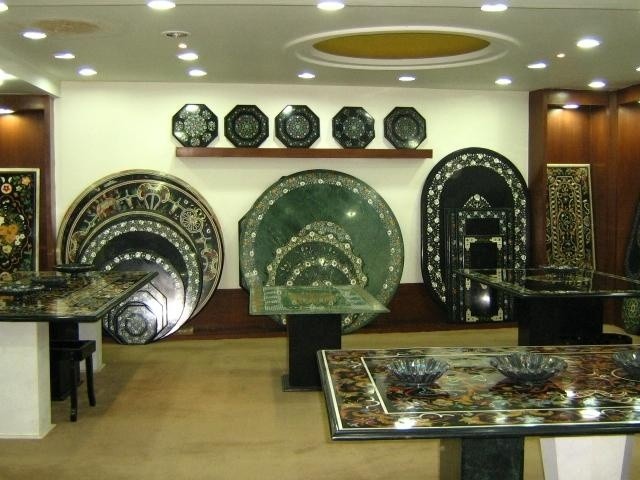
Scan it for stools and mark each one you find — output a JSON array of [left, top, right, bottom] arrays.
[[50, 341, 96, 421]]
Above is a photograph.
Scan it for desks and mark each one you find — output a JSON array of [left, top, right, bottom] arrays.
[[453, 268, 640, 346], [0, 270, 159, 439], [318, 346, 640, 480], [249, 285, 391, 392]]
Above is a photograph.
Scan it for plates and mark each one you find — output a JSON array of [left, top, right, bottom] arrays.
[[331, 106, 375, 149], [172, 104, 218, 147], [54, 168, 225, 345], [611, 349, 640, 378], [224, 104, 269, 149], [238, 168, 404, 336], [489, 353, 570, 382], [419, 147, 531, 328], [383, 106, 427, 150], [275, 105, 320, 148], [383, 358, 450, 386]]
